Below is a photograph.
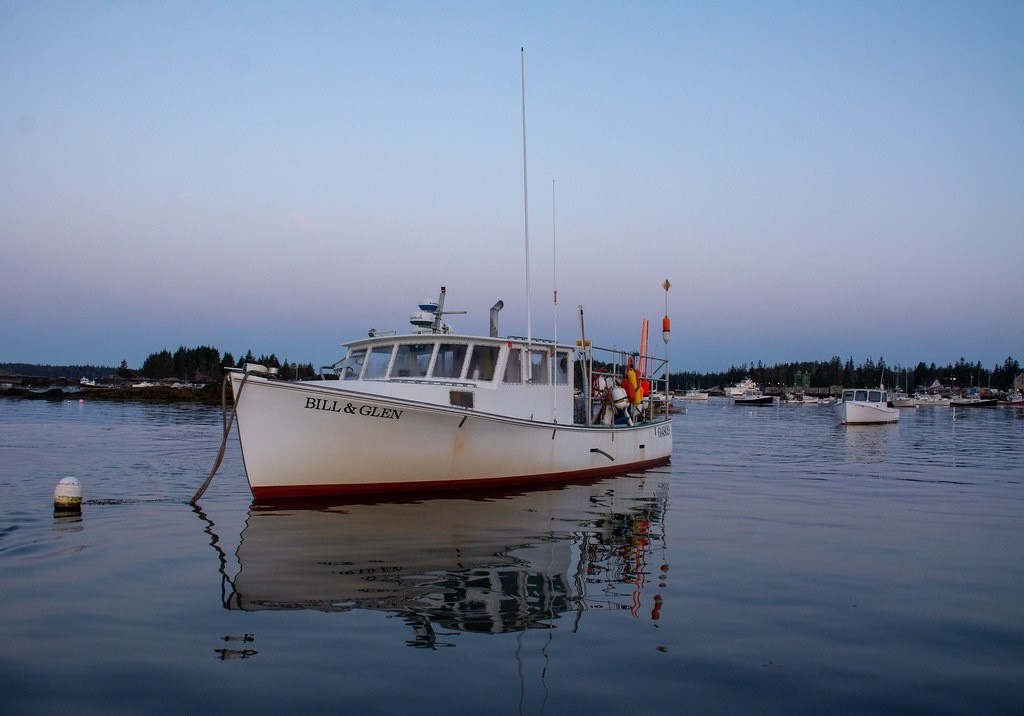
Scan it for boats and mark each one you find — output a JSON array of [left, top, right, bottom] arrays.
[[832, 384, 900, 426], [647, 374, 839, 415], [878, 366, 1024, 413], [221, 42, 673, 506]]
